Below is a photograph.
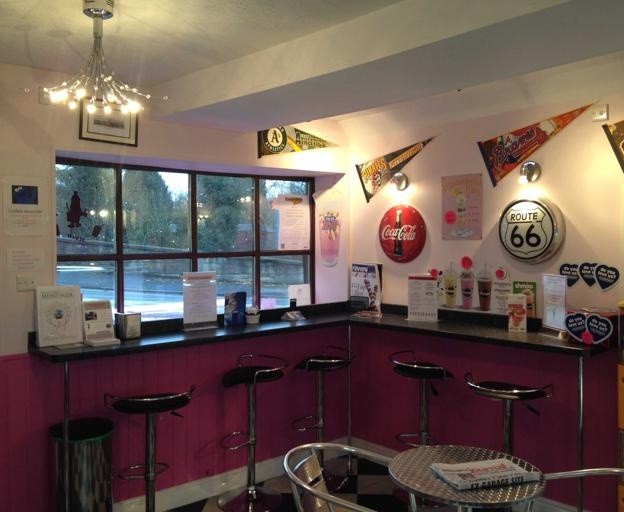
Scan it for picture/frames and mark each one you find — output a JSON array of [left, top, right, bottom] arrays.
[[79, 96, 139, 147]]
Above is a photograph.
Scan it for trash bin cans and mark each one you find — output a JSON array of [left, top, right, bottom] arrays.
[[45, 415, 117, 511]]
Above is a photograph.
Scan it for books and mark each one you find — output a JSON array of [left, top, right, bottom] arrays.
[[36, 285, 122, 347], [350, 263, 384, 319]]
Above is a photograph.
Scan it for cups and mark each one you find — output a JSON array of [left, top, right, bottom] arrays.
[[511, 307, 524, 327], [476, 271, 494, 312], [442, 268, 459, 308], [459, 270, 475, 310], [522, 290, 535, 315], [318, 221, 342, 268]]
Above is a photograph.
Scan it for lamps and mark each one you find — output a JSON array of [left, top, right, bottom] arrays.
[[518, 161, 541, 185], [387, 172, 408, 191], [23, 0, 169, 116]]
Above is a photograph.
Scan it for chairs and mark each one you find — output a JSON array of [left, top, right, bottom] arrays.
[[282, 442, 418, 512], [464, 371, 554, 455], [291, 345, 355, 493], [104, 385, 197, 512], [216, 353, 288, 512], [388, 349, 453, 507]]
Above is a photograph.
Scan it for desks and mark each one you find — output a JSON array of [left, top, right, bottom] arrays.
[[388, 445, 545, 512]]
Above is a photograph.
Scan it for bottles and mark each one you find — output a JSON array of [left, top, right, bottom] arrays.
[[456, 197, 464, 230], [245, 312, 260, 324], [392, 209, 405, 257]]
